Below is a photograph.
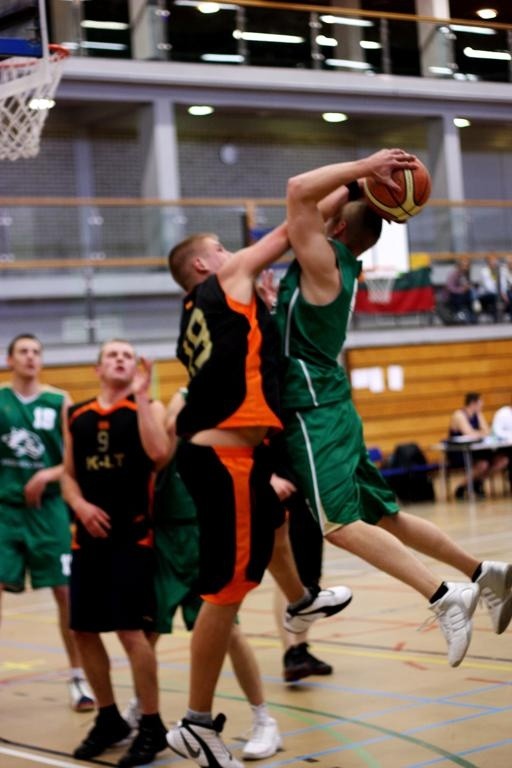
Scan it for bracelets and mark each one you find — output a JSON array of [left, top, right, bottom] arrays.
[[345, 178, 364, 201]]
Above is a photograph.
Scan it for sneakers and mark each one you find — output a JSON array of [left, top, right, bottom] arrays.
[[70, 676, 282, 767], [281, 640, 333, 683], [282, 583, 354, 635], [426, 580, 482, 670], [474, 560, 512, 637]]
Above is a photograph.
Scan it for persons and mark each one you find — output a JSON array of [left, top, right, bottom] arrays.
[[286, 147, 512, 666], [169, 174, 367, 768], [448, 391, 511, 498], [436, 253, 511, 324], [0, 334, 337, 768]]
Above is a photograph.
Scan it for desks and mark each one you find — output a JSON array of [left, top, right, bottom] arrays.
[[431, 439, 512, 505]]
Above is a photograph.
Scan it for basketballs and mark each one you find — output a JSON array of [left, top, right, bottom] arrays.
[[362, 151, 429, 220]]
